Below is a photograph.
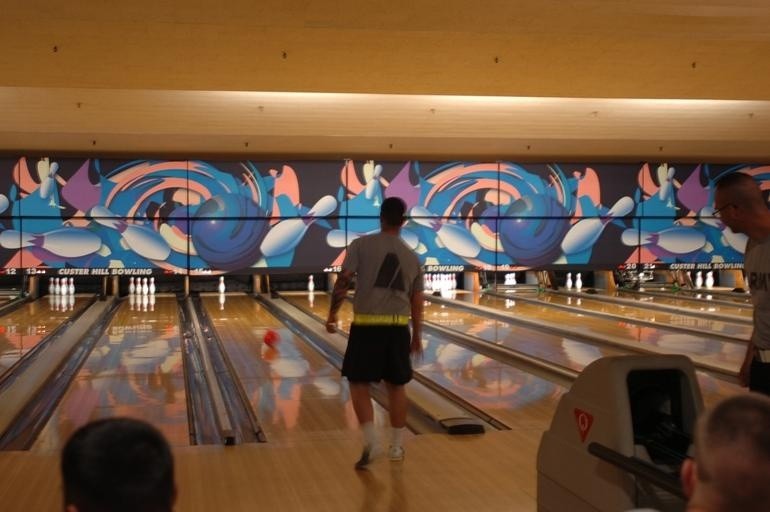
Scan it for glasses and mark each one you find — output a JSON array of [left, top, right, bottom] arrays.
[[713, 203, 736, 219]]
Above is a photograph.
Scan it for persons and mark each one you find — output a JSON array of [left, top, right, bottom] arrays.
[[679, 389, 770, 512], [710, 170, 770, 395], [325, 196, 426, 469], [57, 416, 179, 512]]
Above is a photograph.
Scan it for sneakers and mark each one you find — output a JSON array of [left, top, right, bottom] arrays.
[[389, 445, 405, 462], [356, 441, 385, 467]]
